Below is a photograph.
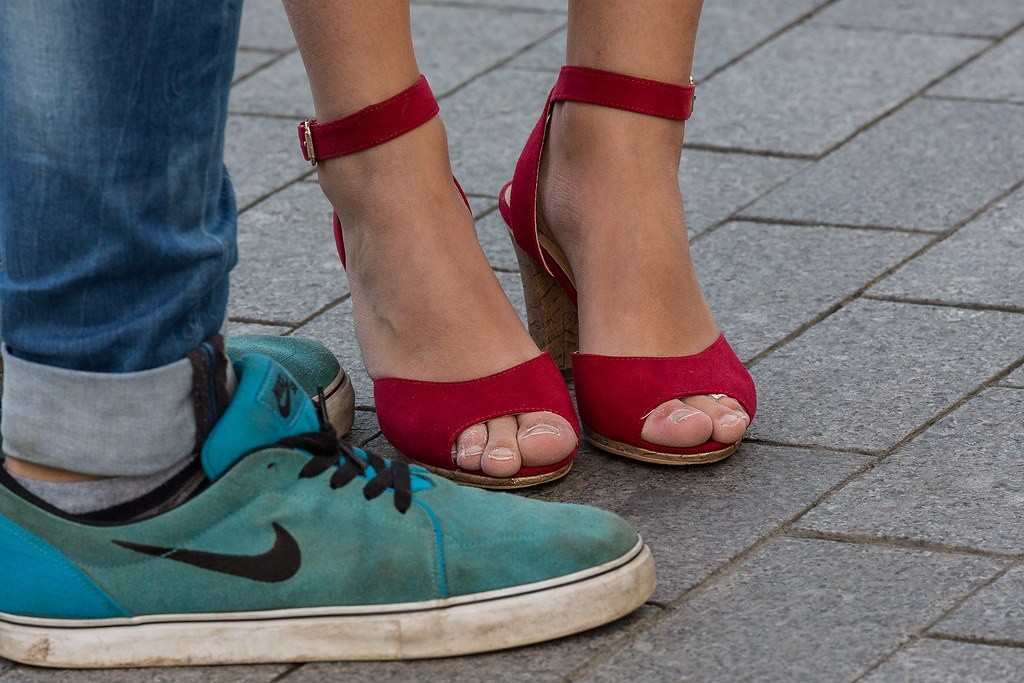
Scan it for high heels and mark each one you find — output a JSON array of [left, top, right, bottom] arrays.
[[298, 75, 580, 490], [498, 66, 758, 465]]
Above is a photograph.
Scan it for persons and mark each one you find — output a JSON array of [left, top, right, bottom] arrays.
[[0, 0, 656, 670], [278, 0, 759, 492]]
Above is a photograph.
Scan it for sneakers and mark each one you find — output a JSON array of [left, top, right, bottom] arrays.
[[223, 334, 354, 439], [0, 353, 656, 670]]
